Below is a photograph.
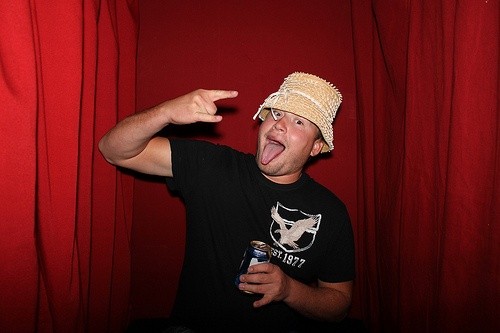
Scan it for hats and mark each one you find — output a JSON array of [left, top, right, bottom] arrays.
[[253, 71, 343, 152]]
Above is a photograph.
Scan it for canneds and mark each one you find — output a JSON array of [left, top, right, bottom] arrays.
[[234, 241, 273, 294]]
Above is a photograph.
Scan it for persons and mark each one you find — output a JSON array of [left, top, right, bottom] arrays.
[[98, 71, 356, 333]]
